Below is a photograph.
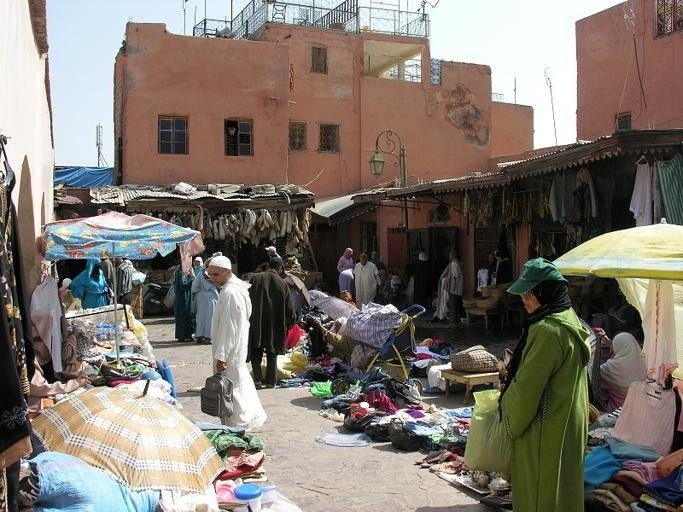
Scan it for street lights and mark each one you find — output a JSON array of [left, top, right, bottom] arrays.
[[369, 129, 411, 269]]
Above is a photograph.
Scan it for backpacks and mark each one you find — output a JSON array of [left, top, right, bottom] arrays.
[[201, 372, 234, 417]]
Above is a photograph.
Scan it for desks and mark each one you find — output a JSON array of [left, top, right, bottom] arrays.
[[465, 307, 505, 329], [440, 369, 508, 405]]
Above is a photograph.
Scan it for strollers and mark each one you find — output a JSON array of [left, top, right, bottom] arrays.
[[330, 304, 426, 395]]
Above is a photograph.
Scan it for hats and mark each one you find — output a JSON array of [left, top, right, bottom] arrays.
[[264, 245, 277, 252], [209, 256, 232, 270], [506, 256, 564, 294]]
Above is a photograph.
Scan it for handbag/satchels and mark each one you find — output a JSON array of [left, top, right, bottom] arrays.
[[200, 368, 235, 419]]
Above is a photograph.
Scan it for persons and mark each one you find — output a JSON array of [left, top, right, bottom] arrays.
[[57, 278, 72, 299], [248, 258, 294, 389], [353, 253, 381, 309], [337, 247, 354, 273], [279, 264, 286, 278], [498, 258, 591, 512], [98, 252, 114, 290], [266, 246, 281, 260], [191, 257, 204, 334], [284, 276, 305, 321], [341, 290, 357, 309], [383, 274, 405, 305], [174, 258, 193, 342], [339, 268, 354, 291], [493, 249, 512, 284], [208, 255, 267, 432], [448, 249, 464, 328], [71, 257, 109, 310], [600, 332, 646, 411], [112, 257, 133, 329], [124, 259, 144, 319], [313, 316, 353, 365], [192, 257, 220, 344]]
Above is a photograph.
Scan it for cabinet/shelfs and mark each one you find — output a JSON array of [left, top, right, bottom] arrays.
[[387, 228, 441, 309]]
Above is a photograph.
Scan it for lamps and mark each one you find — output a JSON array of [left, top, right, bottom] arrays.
[[369, 150, 386, 175]]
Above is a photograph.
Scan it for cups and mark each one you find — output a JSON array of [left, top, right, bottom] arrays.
[[110, 297, 113, 307], [111, 341, 116, 352], [125, 345, 134, 356]]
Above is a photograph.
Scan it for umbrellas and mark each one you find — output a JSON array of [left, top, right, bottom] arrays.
[[284, 271, 311, 308], [32, 379, 226, 496], [309, 289, 329, 300], [550, 217, 683, 385], [38, 210, 205, 273], [312, 297, 358, 322]]
